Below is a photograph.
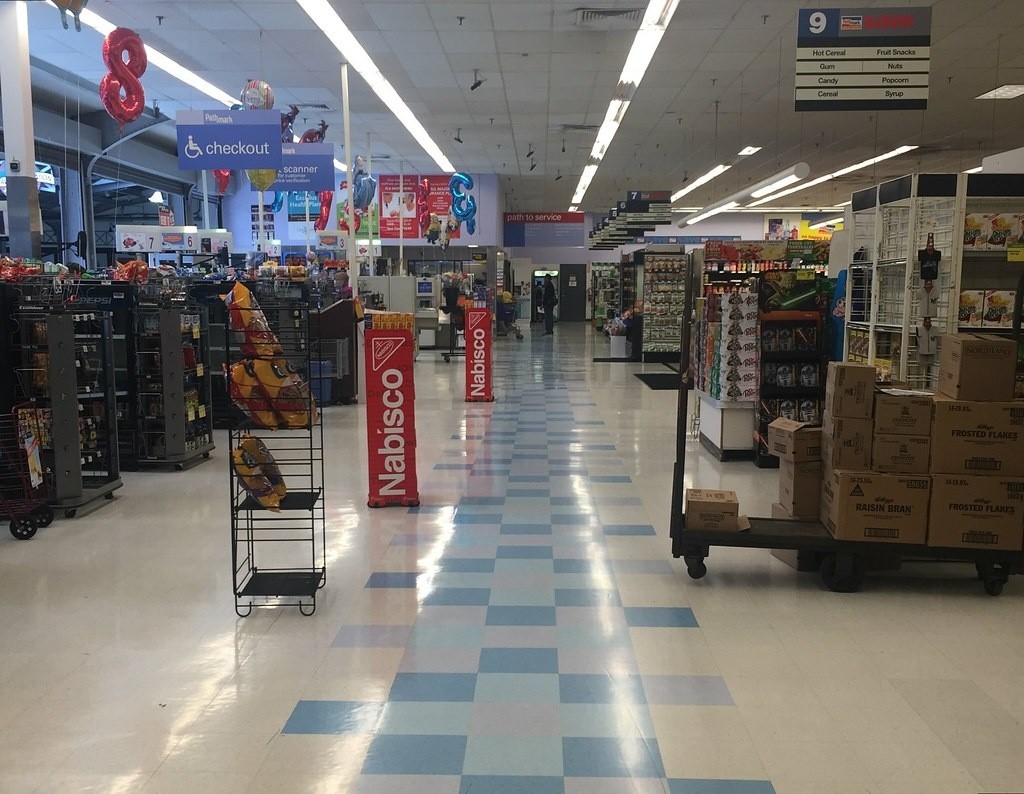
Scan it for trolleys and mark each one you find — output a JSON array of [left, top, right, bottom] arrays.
[[666, 247, 1024, 598], [0, 397, 55, 543]]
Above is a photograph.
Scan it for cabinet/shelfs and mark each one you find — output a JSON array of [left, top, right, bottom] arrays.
[[754, 311, 824, 469], [843, 173, 1024, 392], [692, 247, 828, 310], [0, 264, 328, 617], [591, 242, 692, 363]]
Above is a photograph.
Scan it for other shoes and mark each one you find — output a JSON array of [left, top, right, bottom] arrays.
[[542, 330, 553, 335]]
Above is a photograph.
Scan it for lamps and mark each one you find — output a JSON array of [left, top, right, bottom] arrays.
[[43, 0, 983, 230]]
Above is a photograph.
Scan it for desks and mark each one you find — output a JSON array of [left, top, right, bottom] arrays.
[[694, 388, 756, 462]]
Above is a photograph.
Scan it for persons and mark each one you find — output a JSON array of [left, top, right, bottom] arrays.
[[543, 274, 556, 336], [831, 248, 881, 362], [521, 281, 530, 297], [361, 259, 387, 276], [319, 270, 334, 293], [535, 281, 542, 322], [383, 192, 415, 217], [622, 310, 633, 340], [335, 272, 354, 299]]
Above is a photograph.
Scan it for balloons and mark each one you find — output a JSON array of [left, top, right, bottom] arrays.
[[214, 81, 377, 235], [417, 172, 476, 250], [52, 0, 88, 32], [101, 27, 147, 127]]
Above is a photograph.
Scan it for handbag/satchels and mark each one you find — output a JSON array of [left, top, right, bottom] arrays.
[[552, 299, 558, 306]]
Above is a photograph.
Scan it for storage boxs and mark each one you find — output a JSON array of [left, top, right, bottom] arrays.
[[684, 213, 1024, 551], [465, 300, 487, 309], [364, 313, 416, 351]]
